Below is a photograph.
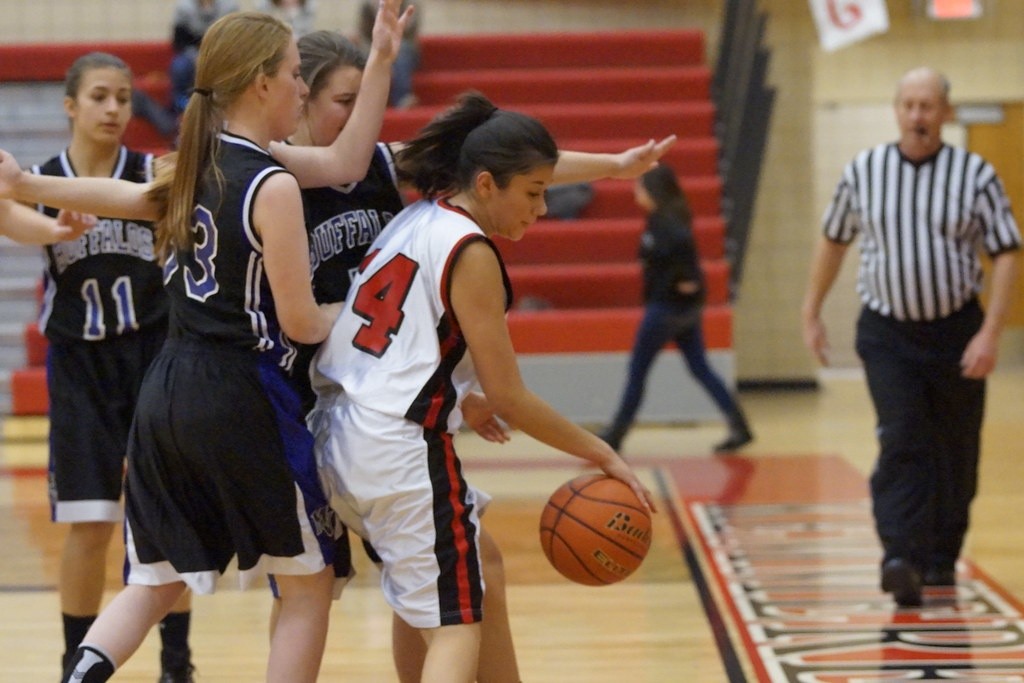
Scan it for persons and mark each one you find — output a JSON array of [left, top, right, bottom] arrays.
[[801, 66, 1024, 607], [358, 0, 418, 107], [1, 56, 198, 683], [601, 162, 753, 453], [170, 0, 240, 110], [259, 0, 315, 37], [304, 91, 657, 683], [63, 0, 414, 683], [1, 31, 677, 683]]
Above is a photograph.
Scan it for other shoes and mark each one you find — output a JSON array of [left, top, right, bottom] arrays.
[[884, 558, 924, 606], [925, 569, 955, 584], [597, 432, 620, 449], [159, 671, 194, 683], [718, 429, 752, 449]]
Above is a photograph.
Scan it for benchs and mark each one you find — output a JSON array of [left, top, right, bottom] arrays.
[[0, 26, 739, 420]]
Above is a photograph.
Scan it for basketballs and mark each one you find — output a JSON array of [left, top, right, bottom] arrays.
[[539, 474, 653, 586]]
